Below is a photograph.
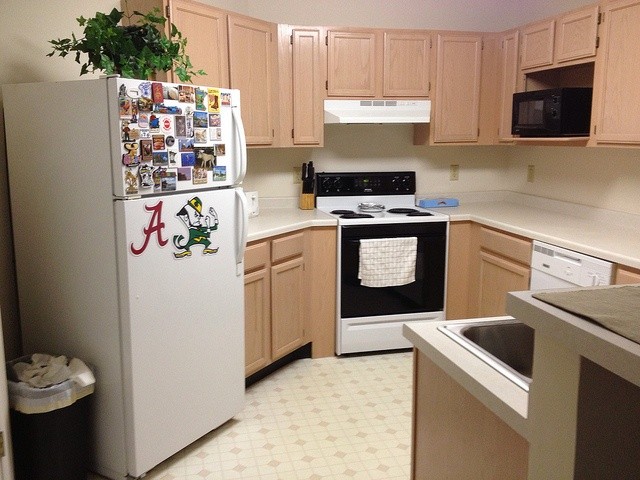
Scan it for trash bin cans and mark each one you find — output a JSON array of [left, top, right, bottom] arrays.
[[5, 353, 96, 479]]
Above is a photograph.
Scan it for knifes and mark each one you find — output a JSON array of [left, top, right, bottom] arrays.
[[300, 161, 315, 210]]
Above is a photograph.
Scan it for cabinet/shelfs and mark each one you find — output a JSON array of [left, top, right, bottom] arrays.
[[588, 0, 640, 151], [414, 26, 481, 148], [471, 223, 531, 335], [492, 26, 522, 146], [121, 1, 284, 153], [245, 231, 306, 377], [505, 4, 594, 145], [290, 23, 327, 150], [325, 29, 432, 101]]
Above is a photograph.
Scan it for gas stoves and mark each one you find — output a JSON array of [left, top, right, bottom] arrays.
[[314, 171, 448, 223]]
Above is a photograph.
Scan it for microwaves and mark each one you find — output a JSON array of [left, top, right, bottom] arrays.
[[509, 88, 592, 135]]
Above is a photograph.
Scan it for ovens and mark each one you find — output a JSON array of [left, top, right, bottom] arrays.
[[335, 223, 448, 356]]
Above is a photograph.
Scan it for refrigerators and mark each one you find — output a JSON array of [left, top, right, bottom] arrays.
[[0, 74, 246, 479]]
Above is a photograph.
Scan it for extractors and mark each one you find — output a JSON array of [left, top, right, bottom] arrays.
[[323, 99, 431, 127]]
